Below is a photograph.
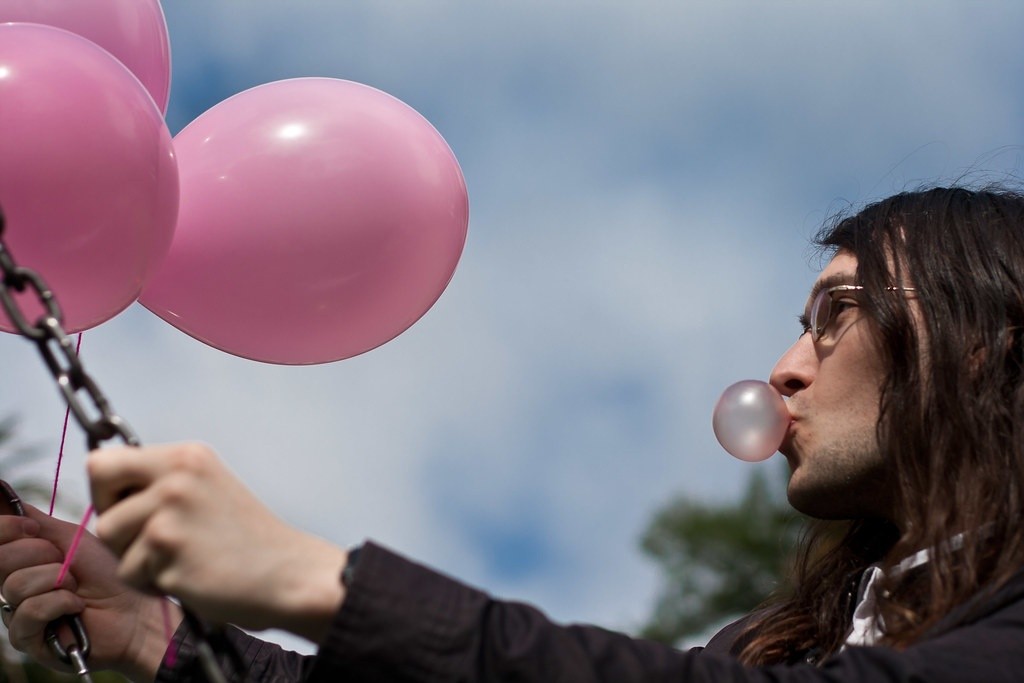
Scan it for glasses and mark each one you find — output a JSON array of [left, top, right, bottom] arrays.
[[798, 280, 922, 343]]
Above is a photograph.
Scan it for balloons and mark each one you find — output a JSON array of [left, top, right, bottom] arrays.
[[0, 0, 469, 365]]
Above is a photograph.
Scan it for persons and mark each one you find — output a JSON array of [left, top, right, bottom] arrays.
[[0, 177, 1023, 683]]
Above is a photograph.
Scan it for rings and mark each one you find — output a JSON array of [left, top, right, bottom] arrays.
[[0, 595, 11, 612]]
